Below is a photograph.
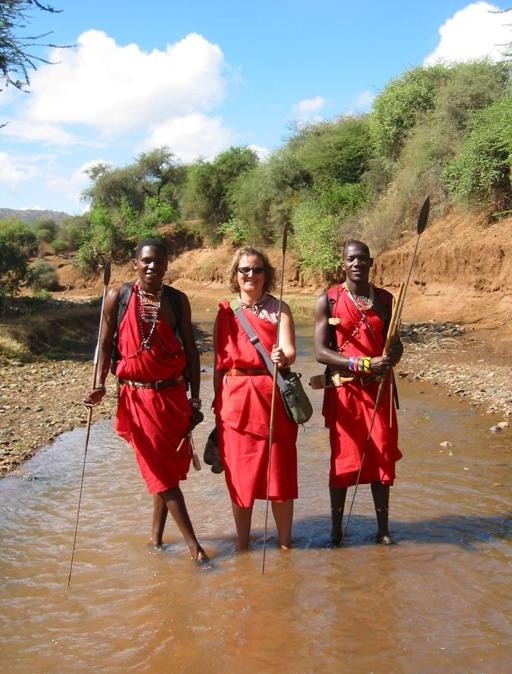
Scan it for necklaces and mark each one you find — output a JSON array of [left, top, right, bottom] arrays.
[[135, 281, 165, 350], [350, 286, 373, 307], [237, 293, 268, 309]]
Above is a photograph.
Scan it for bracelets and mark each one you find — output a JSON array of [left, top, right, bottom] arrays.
[[95, 383, 105, 390], [280, 357, 288, 369], [348, 355, 372, 375], [189, 398, 201, 409]]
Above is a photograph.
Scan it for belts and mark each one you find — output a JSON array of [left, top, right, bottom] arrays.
[[119, 376, 190, 390], [222, 367, 270, 374]]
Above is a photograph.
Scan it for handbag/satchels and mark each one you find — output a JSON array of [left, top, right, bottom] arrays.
[[280, 371, 313, 424]]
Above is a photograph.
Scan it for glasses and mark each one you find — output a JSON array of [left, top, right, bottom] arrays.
[[237, 266, 266, 273]]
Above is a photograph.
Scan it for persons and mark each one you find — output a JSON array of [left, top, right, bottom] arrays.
[[81, 237, 216, 573], [312, 240, 403, 550], [211, 247, 299, 552]]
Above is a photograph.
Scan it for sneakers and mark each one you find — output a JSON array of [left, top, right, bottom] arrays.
[[203, 425, 225, 473]]
